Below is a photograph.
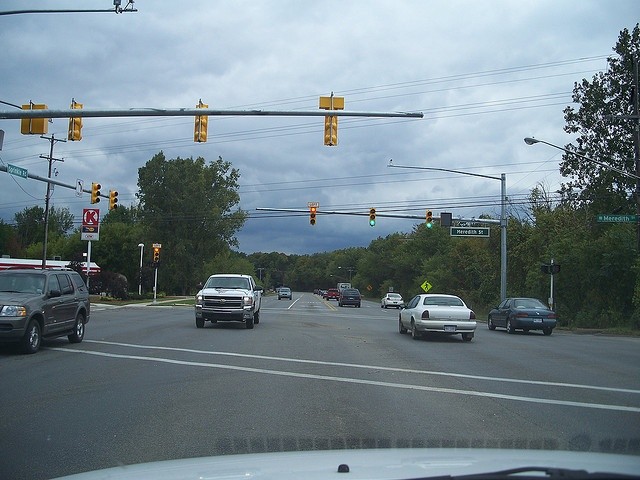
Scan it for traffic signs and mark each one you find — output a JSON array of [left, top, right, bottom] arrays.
[[389, 287, 393, 292], [597, 214, 637, 223], [440, 212, 452, 227], [7, 163, 27, 179], [449, 226, 490, 237], [367, 286, 372, 291]]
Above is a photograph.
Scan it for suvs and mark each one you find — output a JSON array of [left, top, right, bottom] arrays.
[[0, 269, 90, 354]]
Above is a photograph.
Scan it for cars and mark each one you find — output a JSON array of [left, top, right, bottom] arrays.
[[314, 288, 328, 299], [488, 298, 557, 336], [399, 294, 477, 340], [278, 288, 292, 300], [326, 289, 340, 301], [381, 293, 404, 309], [339, 288, 361, 308]]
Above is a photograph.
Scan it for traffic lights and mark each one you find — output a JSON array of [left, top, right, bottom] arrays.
[[426, 210, 433, 228], [543, 264, 561, 274], [369, 208, 376, 227], [310, 207, 316, 225], [154, 248, 159, 262], [91, 182, 101, 204], [109, 190, 118, 210]]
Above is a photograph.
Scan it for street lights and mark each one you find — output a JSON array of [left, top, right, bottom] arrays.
[[330, 275, 345, 279], [524, 136, 640, 179], [338, 267, 352, 280], [137, 243, 145, 295]]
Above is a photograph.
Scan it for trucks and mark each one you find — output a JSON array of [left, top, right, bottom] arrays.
[[337, 282, 351, 292]]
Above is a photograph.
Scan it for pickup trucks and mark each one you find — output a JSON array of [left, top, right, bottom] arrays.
[[195, 274, 264, 329]]
